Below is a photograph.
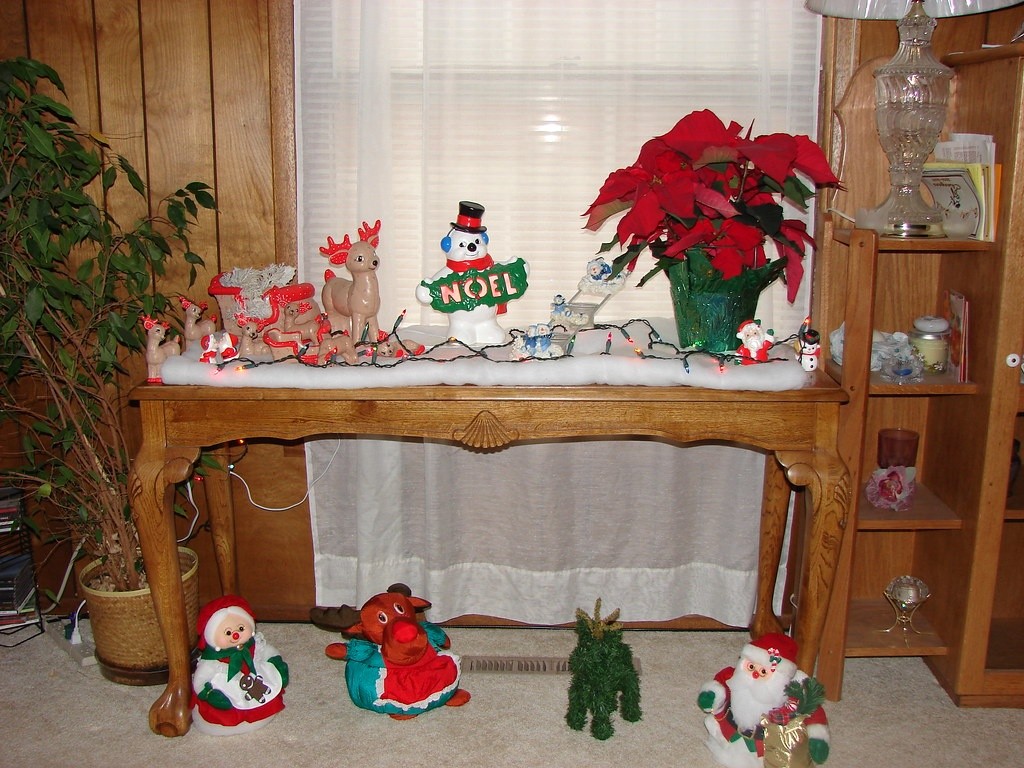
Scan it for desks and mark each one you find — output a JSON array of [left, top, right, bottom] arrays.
[[127, 366, 849, 738]]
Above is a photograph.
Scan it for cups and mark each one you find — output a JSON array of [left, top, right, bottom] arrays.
[[877, 427, 920, 470]]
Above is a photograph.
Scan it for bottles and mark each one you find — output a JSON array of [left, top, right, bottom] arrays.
[[908, 328, 951, 376]]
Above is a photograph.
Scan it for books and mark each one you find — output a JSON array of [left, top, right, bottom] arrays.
[[920, 133, 1002, 242]]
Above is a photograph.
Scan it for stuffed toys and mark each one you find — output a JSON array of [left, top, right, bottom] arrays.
[[310, 583, 472, 719], [696, 633, 830, 768], [190, 595, 290, 735]]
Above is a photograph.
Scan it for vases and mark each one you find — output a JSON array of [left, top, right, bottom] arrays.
[[668, 250, 787, 352]]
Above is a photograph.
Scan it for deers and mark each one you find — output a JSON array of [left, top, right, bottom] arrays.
[[140, 219, 425, 383]]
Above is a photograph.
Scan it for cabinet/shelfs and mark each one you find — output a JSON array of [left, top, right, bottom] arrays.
[[810, 3, 1024, 706]]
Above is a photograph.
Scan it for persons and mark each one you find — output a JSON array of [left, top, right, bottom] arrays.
[[736, 320, 772, 365]]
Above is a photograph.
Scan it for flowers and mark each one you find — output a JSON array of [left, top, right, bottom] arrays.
[[580, 108, 846, 303]]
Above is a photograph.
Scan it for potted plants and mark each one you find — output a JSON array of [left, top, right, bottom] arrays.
[[0, 56, 227, 682]]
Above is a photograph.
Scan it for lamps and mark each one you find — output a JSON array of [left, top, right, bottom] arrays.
[[805, 0, 1023, 240]]
